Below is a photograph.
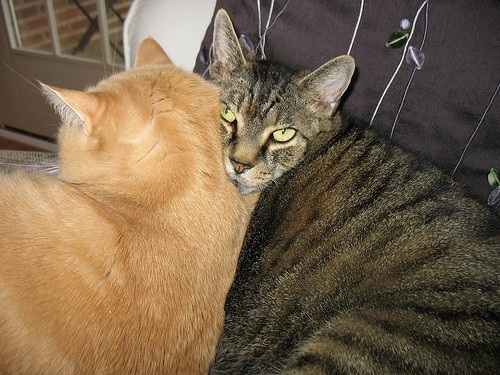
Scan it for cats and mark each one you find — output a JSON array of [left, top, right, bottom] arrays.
[[206, 9, 500, 375], [0, 36, 262, 375]]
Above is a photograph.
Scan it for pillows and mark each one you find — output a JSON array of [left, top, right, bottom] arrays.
[[190, 0, 500, 219]]
